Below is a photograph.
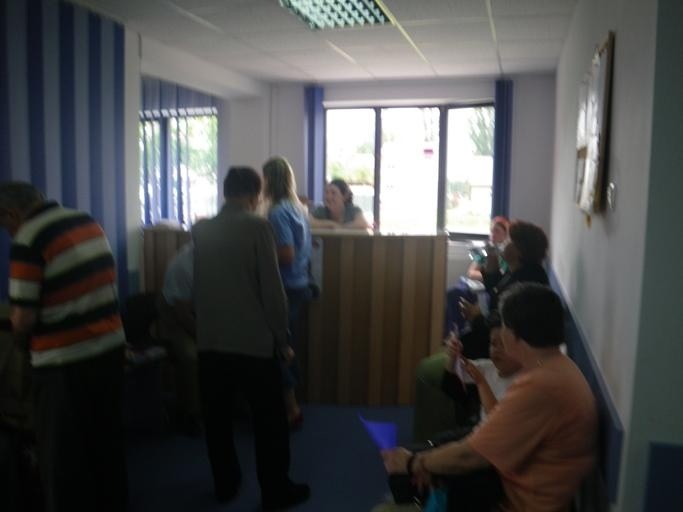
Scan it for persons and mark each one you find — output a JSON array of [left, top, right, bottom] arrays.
[[311, 179, 367, 233], [0, 181, 130, 504]]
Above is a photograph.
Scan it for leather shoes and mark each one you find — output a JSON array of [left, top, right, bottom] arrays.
[[216, 475, 311, 507]]
